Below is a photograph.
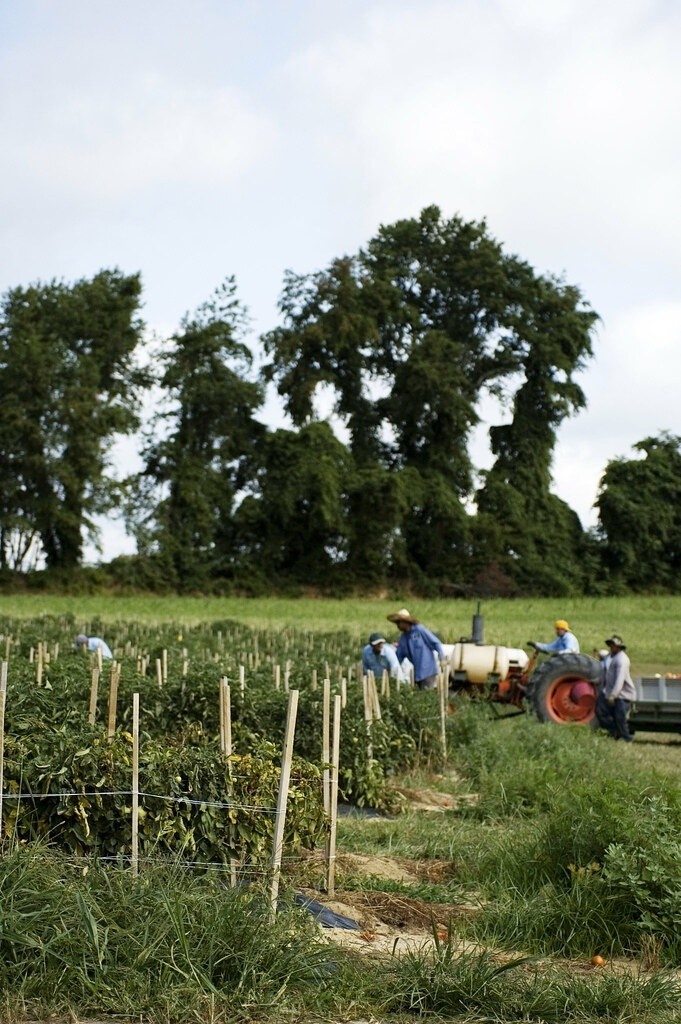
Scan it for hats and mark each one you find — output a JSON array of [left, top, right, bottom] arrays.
[[555, 620, 571, 632], [604, 634, 626, 650], [387, 608, 418, 625], [76, 634, 87, 644], [370, 633, 386, 645]]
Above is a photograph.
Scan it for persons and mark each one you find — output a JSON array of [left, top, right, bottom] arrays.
[[75, 633, 113, 658], [532, 620, 580, 655], [395, 608, 446, 690], [595, 635, 637, 743], [362, 633, 403, 683]]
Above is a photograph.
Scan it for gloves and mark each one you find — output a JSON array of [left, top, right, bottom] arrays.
[[608, 696, 614, 703]]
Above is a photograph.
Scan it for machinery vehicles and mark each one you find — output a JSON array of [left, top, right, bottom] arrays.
[[436, 602, 605, 731]]
